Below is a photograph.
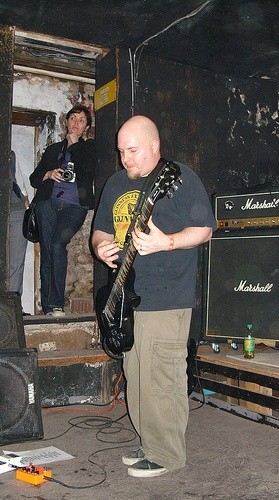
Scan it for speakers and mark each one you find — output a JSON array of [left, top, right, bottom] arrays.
[[0, 348, 45, 445], [204, 228, 279, 351], [0, 290, 26, 348]]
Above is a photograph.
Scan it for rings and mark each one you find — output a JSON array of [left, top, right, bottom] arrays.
[[140, 245, 142, 251]]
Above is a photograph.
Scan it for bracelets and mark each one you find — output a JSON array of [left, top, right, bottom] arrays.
[[168, 235, 174, 250]]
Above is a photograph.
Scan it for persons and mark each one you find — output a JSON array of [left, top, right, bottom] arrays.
[[8, 150, 31, 316], [89, 116, 217, 478], [29, 105, 96, 316]]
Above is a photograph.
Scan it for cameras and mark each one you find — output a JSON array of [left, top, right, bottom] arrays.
[[61, 170, 76, 183]]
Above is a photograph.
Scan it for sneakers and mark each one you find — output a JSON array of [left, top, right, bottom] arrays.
[[121, 446, 146, 466], [128, 458, 169, 478]]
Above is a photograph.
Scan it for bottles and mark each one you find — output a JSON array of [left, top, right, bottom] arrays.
[[243, 324, 256, 359]]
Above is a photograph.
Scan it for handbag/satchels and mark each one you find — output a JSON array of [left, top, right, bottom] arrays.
[[22, 208, 39, 243]]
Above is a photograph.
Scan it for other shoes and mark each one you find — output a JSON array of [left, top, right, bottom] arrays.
[[53, 307, 66, 317], [46, 312, 54, 316]]
